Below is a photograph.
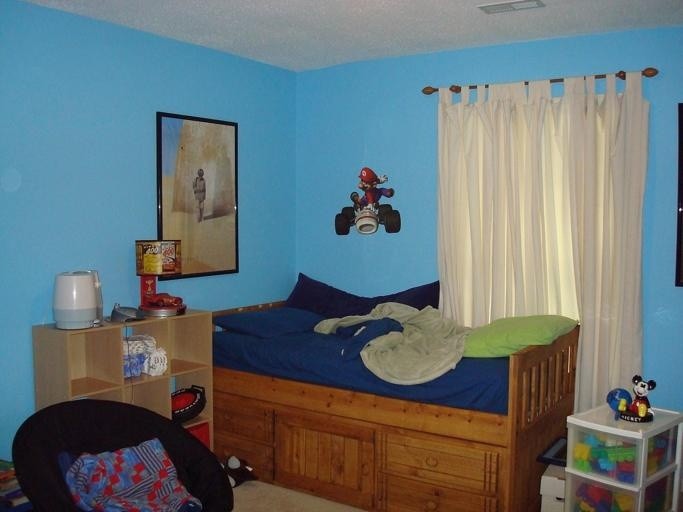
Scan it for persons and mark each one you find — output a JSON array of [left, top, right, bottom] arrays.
[[192, 169, 206, 223]]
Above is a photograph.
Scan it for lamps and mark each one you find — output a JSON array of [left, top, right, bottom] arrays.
[[133, 238, 186, 318]]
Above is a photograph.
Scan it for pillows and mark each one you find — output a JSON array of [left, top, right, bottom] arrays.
[[462, 315, 576, 357]]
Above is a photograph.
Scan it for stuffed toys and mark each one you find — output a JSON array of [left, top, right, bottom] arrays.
[[221, 456, 258, 488]]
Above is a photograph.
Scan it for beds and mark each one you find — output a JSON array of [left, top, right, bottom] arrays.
[[211, 299, 580, 511]]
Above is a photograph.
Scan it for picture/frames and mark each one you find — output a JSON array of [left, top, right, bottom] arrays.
[[673, 102, 683, 287], [155, 112, 239, 282]]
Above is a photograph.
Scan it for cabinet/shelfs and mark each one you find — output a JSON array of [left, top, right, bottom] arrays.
[[536, 401, 682, 512], [31, 307, 212, 458]]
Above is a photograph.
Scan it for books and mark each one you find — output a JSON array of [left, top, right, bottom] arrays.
[[0, 459, 34, 512]]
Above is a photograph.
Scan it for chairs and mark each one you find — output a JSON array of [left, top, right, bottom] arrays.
[[11, 399, 233, 511]]
